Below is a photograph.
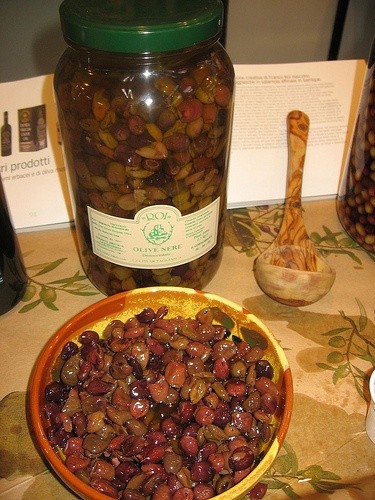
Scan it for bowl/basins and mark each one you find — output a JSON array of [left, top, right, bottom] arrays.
[[28, 284, 293, 500]]
